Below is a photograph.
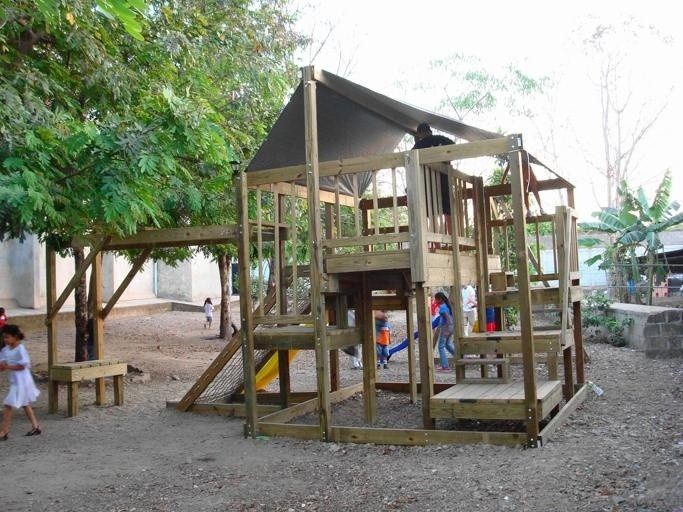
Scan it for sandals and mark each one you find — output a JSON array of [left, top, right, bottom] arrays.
[[0, 430, 11, 439], [26, 426, 42, 437]]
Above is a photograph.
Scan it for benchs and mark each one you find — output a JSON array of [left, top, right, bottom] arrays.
[[48, 355, 129, 419]]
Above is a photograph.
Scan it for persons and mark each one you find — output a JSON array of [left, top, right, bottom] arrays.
[[429, 296, 437, 322], [0, 324, 42, 443], [202, 297, 215, 330], [461, 285, 478, 336], [374, 310, 393, 370], [433, 292, 455, 371], [0, 306, 8, 352], [411, 122, 456, 250], [470, 302, 495, 332], [345, 308, 363, 370]]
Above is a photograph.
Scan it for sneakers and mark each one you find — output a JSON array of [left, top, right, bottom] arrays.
[[435, 366, 452, 372], [376, 363, 390, 370]]
